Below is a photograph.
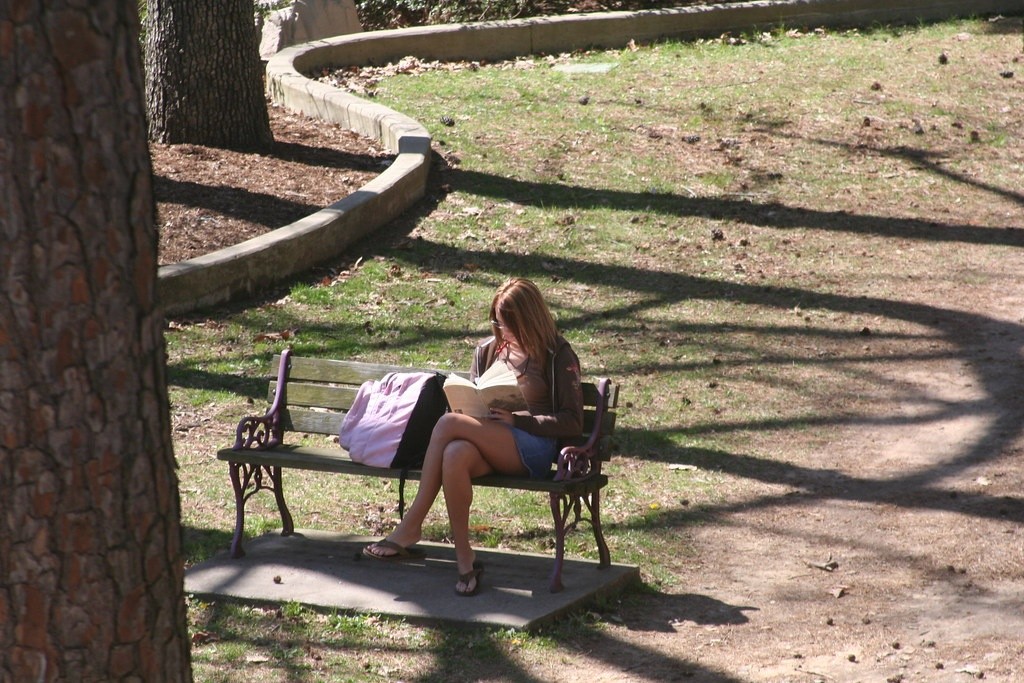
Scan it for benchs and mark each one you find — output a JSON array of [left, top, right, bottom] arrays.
[[217, 350, 621, 592]]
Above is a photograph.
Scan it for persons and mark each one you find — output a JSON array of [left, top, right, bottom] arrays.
[[361, 275, 585, 597]]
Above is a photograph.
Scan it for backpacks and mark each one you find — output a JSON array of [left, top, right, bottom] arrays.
[[339, 372, 453, 471]]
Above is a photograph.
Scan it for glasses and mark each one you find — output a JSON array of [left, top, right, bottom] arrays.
[[491, 320, 508, 329]]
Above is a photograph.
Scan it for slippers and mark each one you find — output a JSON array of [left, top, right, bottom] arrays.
[[456, 560, 485, 596], [363, 539, 421, 563]]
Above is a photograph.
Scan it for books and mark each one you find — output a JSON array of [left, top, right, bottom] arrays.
[[442, 359, 531, 417]]
